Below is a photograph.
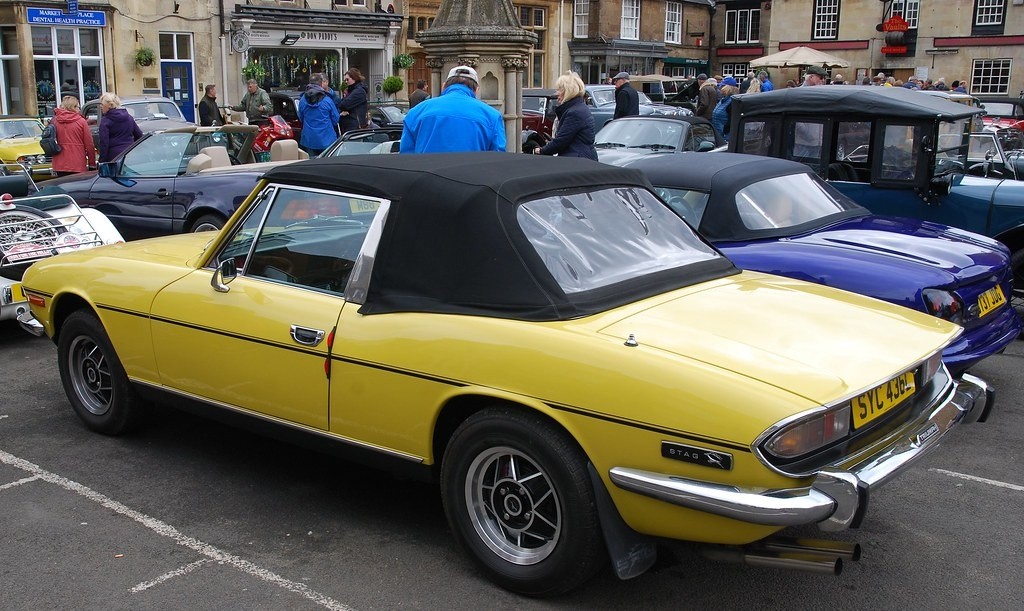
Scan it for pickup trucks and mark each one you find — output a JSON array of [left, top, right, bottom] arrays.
[[28, 128, 327, 234]]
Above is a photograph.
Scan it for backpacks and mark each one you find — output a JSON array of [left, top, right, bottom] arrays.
[[41, 115, 62, 156]]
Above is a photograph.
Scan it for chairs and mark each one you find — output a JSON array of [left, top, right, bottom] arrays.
[[198, 146, 234, 171], [270, 139, 300, 163], [830, 164, 858, 182]]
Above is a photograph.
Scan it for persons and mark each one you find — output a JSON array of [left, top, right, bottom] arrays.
[[297, 68, 369, 156], [679, 75, 696, 102], [697, 71, 773, 141], [399, 66, 507, 153], [48, 96, 96, 176], [229, 79, 272, 125], [613, 72, 639, 119], [535, 75, 598, 162], [787, 65, 845, 159], [199, 84, 223, 126], [862, 72, 967, 105], [98, 92, 142, 163], [408, 79, 431, 109]]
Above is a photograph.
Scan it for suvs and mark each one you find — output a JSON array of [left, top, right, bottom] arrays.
[[75, 94, 201, 154], [520, 87, 564, 137]]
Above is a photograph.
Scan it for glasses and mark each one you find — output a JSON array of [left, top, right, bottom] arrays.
[[616, 79, 618, 82], [345, 79, 349, 82]]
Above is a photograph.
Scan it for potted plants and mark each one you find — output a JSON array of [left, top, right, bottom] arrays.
[[241, 64, 266, 87], [134, 48, 157, 67], [396, 53, 416, 67], [383, 76, 405, 93]]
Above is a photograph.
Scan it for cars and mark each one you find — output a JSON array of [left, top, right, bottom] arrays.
[[573, 85, 733, 154], [0, 115, 100, 183], [314, 129, 406, 156], [712, 88, 1023, 245], [12, 154, 999, 603], [0, 165, 125, 336], [595, 151, 1022, 377], [366, 106, 415, 128], [262, 91, 304, 120]]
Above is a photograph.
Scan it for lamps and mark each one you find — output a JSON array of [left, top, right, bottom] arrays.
[[282, 33, 299, 46]]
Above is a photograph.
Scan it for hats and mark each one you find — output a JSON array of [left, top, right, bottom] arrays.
[[714, 74, 724, 80], [447, 66, 478, 84], [613, 72, 631, 79], [696, 73, 708, 80], [717, 76, 737, 86], [205, 85, 215, 89], [806, 66, 830, 76]]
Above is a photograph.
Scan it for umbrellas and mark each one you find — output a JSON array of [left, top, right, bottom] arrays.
[[749, 45, 850, 82]]
